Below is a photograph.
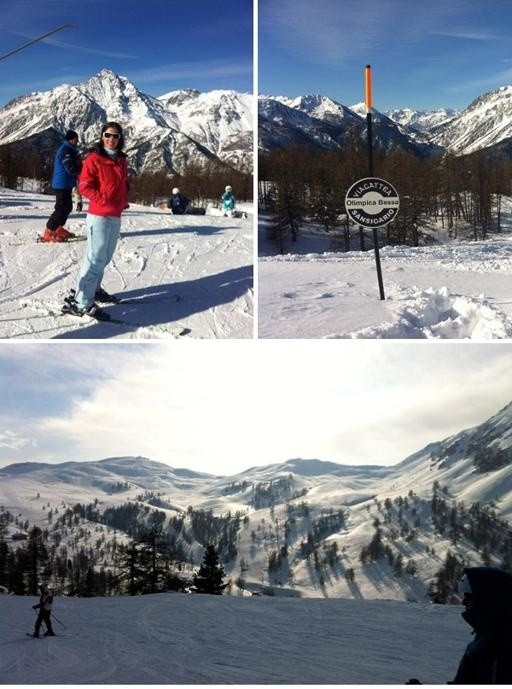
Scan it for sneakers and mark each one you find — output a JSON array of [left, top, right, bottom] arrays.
[[43, 225, 75, 242], [76, 202, 83, 211], [72, 301, 111, 322], [94, 288, 116, 302]]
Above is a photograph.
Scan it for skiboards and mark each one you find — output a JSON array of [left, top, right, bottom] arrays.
[[36, 229, 123, 242], [63, 288, 184, 336], [27, 632, 71, 640]]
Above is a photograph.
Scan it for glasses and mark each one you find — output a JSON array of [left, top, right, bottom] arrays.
[[102, 132, 120, 140]]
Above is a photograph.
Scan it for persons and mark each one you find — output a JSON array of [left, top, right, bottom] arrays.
[[167, 187, 206, 214], [222, 185, 247, 218], [62, 122, 129, 322], [44, 130, 82, 242], [32, 584, 55, 637], [406, 566, 512, 685], [72, 186, 84, 211]]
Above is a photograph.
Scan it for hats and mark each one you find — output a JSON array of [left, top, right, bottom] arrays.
[[65, 130, 78, 142]]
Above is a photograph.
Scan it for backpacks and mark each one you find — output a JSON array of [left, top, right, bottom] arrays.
[[187, 208, 206, 215], [171, 197, 186, 214]]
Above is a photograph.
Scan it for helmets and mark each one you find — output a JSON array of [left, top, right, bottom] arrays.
[[171, 187, 180, 195], [224, 185, 232, 192]]
